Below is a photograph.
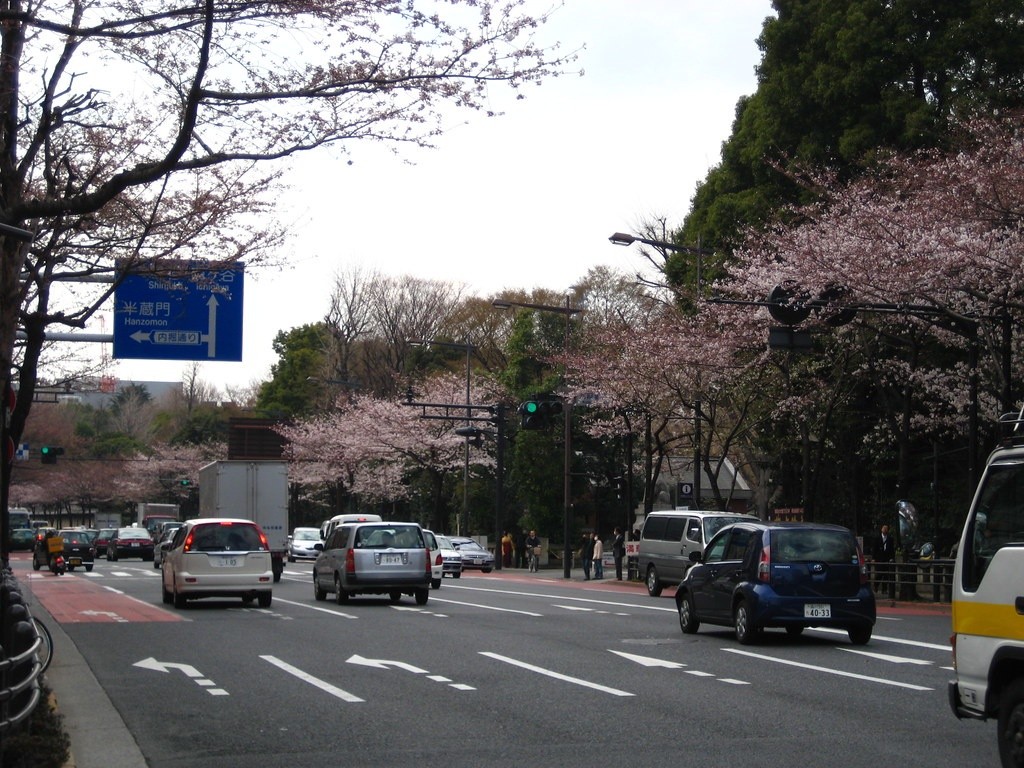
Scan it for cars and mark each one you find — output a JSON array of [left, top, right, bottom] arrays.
[[6, 502, 187, 561], [33, 530, 94, 571], [161, 517, 274, 608], [673, 520, 877, 645], [362, 528, 444, 589], [436, 535, 463, 579], [287, 527, 325, 561], [447, 535, 494, 575]]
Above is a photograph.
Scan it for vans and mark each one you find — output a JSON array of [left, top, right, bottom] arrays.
[[637, 509, 766, 598], [318, 513, 382, 548]]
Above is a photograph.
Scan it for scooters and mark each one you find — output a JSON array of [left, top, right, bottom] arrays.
[[46, 552, 68, 576]]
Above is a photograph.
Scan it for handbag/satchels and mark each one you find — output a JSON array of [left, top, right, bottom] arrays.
[[621, 548, 626, 556]]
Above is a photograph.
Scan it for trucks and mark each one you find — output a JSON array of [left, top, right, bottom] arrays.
[[197, 459, 290, 582], [893, 409, 1024, 768]]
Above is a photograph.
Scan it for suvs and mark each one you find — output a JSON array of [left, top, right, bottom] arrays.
[[311, 521, 432, 605]]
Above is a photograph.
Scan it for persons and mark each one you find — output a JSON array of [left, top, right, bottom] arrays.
[[871, 526, 895, 594], [502, 529, 541, 569], [632, 529, 641, 578], [611, 528, 624, 580], [583, 532, 603, 580]]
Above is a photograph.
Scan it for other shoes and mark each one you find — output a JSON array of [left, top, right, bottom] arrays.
[[584, 579, 590, 581], [592, 577, 603, 580]]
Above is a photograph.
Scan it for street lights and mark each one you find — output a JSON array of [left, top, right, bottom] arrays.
[[609, 230, 704, 510], [407, 337, 478, 539], [488, 298, 582, 579]]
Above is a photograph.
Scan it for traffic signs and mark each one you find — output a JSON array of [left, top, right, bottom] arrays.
[[111, 252, 246, 361]]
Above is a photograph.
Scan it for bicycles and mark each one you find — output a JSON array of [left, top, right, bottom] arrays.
[[528, 544, 542, 573]]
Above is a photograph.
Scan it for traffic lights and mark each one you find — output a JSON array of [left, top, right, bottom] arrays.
[[519, 401, 563, 415], [181, 480, 189, 486], [523, 415, 543, 429], [41, 447, 64, 456]]
